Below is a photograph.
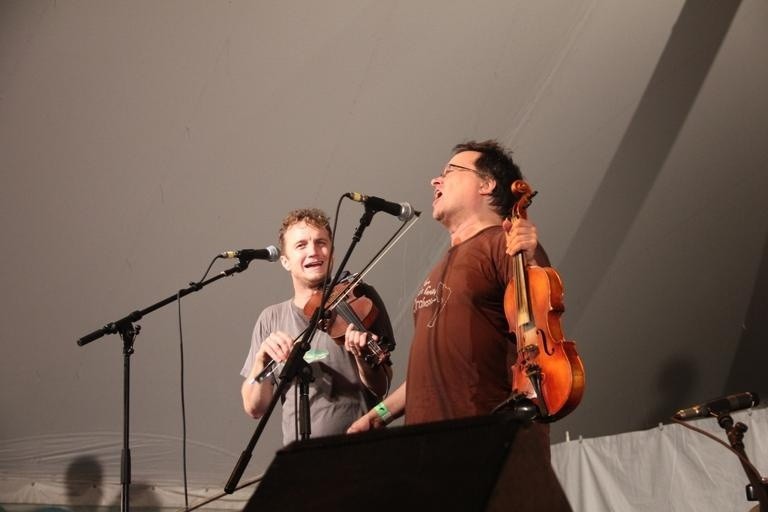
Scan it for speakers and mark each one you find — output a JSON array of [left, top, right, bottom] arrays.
[[240, 413, 576, 512]]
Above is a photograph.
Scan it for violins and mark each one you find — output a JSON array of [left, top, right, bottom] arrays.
[[502, 180, 587, 422], [303, 279, 391, 367]]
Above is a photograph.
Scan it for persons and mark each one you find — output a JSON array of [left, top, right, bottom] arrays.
[[239, 209, 392, 449], [347, 141, 574, 512]]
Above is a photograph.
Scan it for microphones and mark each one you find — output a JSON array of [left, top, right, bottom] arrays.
[[676, 391, 760, 420], [220, 245, 281, 262], [346, 191, 415, 221]]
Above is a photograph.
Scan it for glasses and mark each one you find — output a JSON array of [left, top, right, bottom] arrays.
[[442, 163, 492, 178]]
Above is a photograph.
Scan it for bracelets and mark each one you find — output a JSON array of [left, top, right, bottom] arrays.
[[373, 402, 394, 425]]
